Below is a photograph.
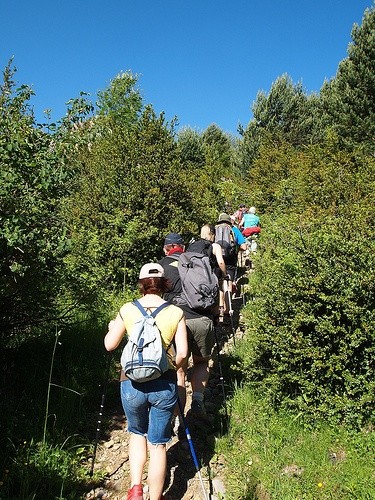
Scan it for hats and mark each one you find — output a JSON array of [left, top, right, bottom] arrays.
[[138, 263, 164, 279], [163, 233, 184, 244], [217, 212, 231, 223]]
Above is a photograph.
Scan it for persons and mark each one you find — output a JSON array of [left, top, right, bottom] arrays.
[[104, 263, 188, 500], [186, 204, 261, 382], [157, 233, 219, 434]]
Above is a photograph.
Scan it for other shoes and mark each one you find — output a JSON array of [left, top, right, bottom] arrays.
[[223, 316, 230, 325], [218, 309, 224, 316], [171, 418, 185, 437], [126, 484, 143, 500], [190, 399, 206, 418]]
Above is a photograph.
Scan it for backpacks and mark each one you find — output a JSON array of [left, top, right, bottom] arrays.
[[119, 300, 171, 383], [213, 222, 237, 256], [186, 238, 215, 270], [165, 251, 220, 309]]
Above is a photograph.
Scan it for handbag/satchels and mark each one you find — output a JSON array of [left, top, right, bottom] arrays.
[[242, 227, 260, 236]]
